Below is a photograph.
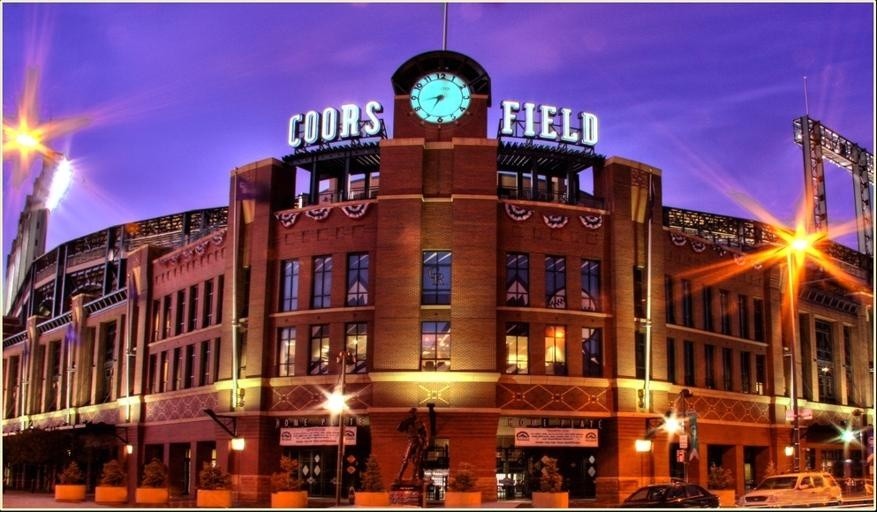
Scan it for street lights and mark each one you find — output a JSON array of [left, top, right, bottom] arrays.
[[334, 350, 358, 507], [786, 235, 807, 473], [34, 152, 76, 209]]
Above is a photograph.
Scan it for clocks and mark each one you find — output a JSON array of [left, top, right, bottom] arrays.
[[409, 72, 473, 127]]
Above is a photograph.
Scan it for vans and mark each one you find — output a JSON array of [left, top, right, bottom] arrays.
[[736, 471, 843, 509]]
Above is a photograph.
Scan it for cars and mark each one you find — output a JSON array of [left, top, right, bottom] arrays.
[[608, 481, 719, 509]]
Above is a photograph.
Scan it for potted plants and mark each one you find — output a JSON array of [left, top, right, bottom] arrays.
[[53, 461, 85, 502], [444, 464, 484, 509], [352, 457, 389, 508], [134, 464, 172, 504], [197, 467, 236, 508], [531, 455, 569, 509], [95, 462, 128, 503], [268, 466, 309, 508]]
